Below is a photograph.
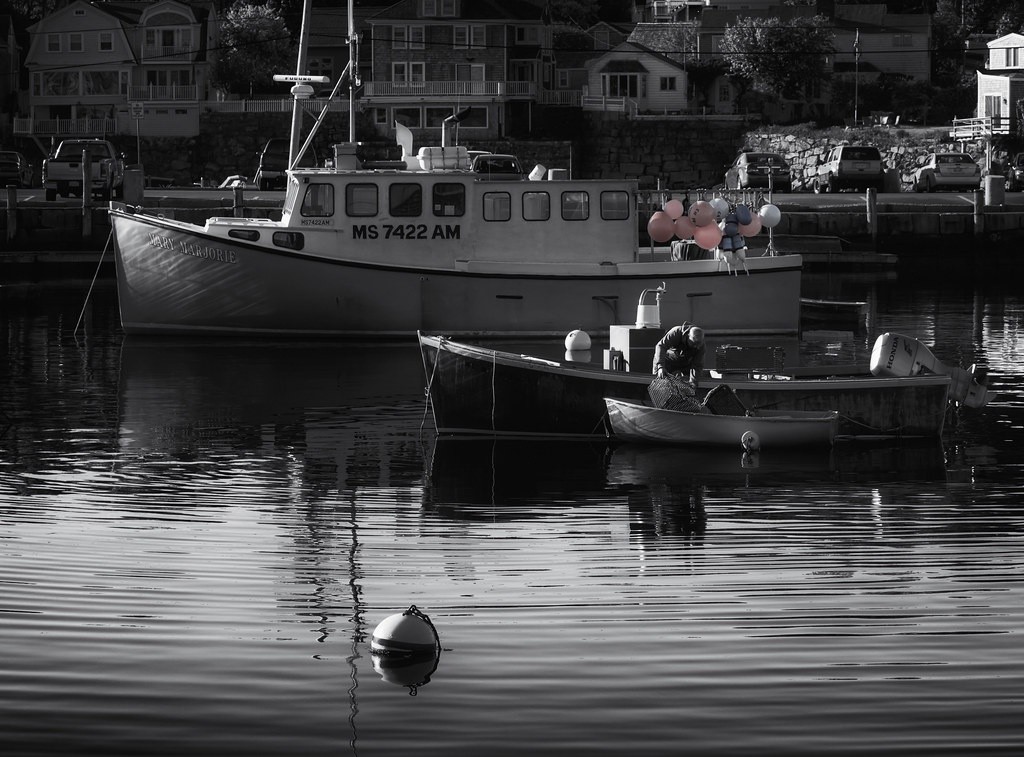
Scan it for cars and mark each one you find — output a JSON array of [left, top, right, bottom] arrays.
[[1007, 153, 1023, 191], [0, 151, 36, 189], [722, 153, 791, 193], [313, 89, 347, 102], [911, 152, 981, 192]]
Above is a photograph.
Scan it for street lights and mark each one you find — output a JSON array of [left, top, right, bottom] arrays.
[[853, 29, 861, 126]]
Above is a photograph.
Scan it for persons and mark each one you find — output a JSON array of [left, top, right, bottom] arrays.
[[653, 324, 706, 388]]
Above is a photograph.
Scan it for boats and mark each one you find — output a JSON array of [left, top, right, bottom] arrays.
[[604, 386, 840, 445], [638, 235, 902, 290], [414, 285, 994, 439], [102, 0, 806, 336]]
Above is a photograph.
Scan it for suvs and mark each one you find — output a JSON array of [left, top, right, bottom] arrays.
[[812, 146, 885, 193], [254, 137, 320, 191]]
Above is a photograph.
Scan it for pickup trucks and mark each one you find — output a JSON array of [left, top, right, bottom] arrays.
[[43, 139, 126, 200]]
